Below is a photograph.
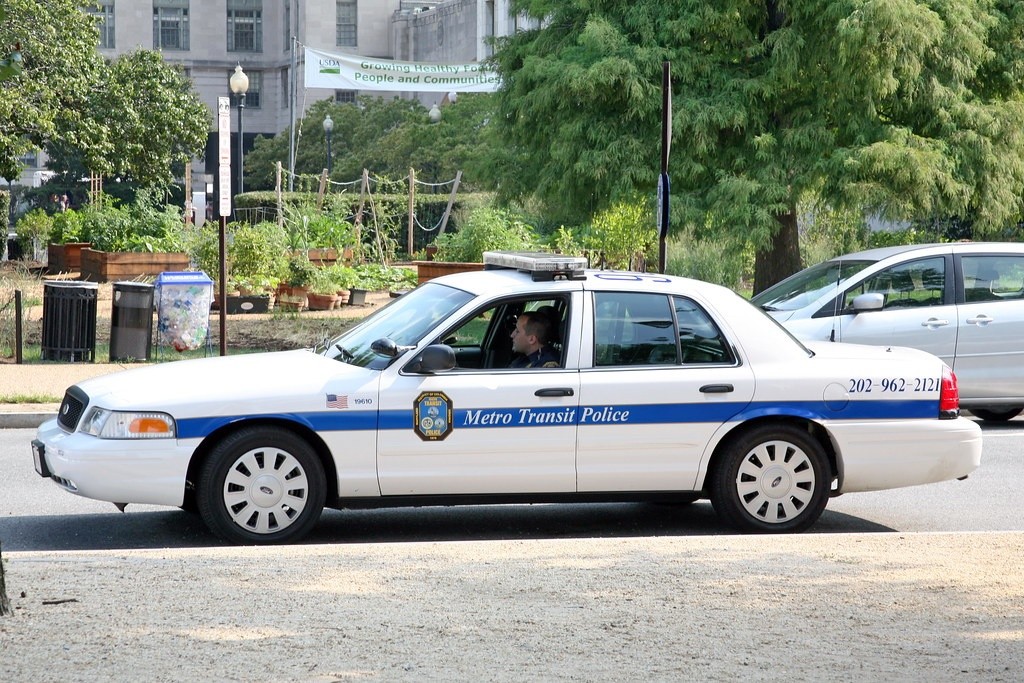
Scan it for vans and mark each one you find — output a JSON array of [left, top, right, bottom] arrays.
[[708, 240, 1024, 421]]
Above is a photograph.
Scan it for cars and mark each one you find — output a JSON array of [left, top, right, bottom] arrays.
[[32, 253, 980, 527]]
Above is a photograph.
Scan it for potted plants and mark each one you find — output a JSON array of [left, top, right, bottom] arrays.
[[6, 185, 193, 283], [191, 187, 369, 314]]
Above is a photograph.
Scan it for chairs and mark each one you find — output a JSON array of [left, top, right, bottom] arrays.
[[920, 267, 941, 305], [889, 269, 918, 309], [537, 305, 560, 346]]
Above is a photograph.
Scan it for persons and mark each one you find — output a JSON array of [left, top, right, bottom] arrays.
[[511, 310, 562, 368], [47, 193, 70, 214]]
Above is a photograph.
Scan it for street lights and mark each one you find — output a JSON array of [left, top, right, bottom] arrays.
[[428, 104, 441, 223], [230, 63, 250, 191], [322, 115, 334, 173]]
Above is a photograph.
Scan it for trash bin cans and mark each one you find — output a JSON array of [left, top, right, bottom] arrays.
[[42, 280, 99, 362], [157, 272, 214, 353], [108, 283, 155, 363], [7, 237, 34, 261]]
